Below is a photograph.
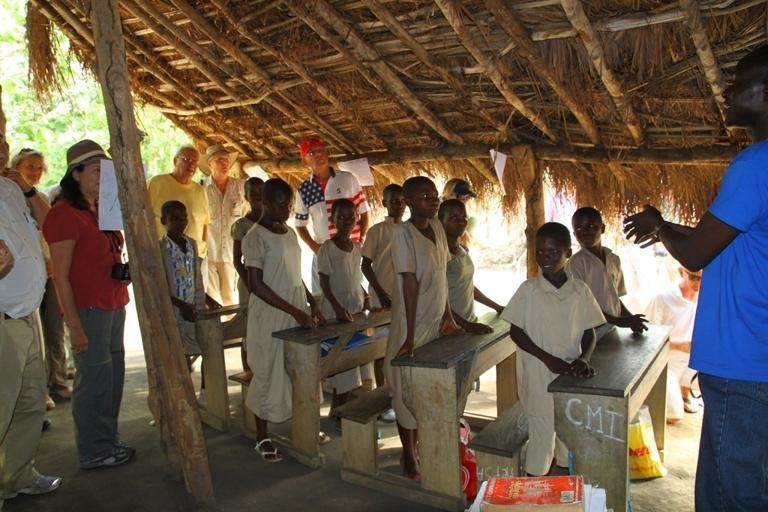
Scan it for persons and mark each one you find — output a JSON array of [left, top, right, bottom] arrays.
[[622, 40, 766, 511], [149, 135, 704, 483], [0, 111, 137, 503]]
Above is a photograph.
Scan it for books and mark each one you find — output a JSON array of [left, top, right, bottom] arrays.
[[464, 475, 614, 512]]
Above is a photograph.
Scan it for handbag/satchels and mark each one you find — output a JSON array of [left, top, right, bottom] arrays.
[[630, 405, 668, 483]]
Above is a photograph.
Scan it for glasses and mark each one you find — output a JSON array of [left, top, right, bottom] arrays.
[[686, 270, 701, 281]]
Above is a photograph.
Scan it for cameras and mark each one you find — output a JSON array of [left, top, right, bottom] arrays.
[[113, 263, 130, 280]]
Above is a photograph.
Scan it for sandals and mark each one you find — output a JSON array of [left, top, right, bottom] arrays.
[[319, 431, 330, 444], [254, 439, 282, 463]]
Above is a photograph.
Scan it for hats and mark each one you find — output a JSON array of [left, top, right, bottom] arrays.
[[61, 140, 112, 190], [300, 135, 329, 157], [199, 144, 239, 176], [445, 178, 475, 197]]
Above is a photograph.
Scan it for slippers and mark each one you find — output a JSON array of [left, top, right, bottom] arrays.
[[682, 397, 698, 413]]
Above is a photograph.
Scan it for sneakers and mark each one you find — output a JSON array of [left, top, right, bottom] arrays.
[[81, 447, 135, 470], [17, 475, 62, 495], [43, 369, 75, 427]]
